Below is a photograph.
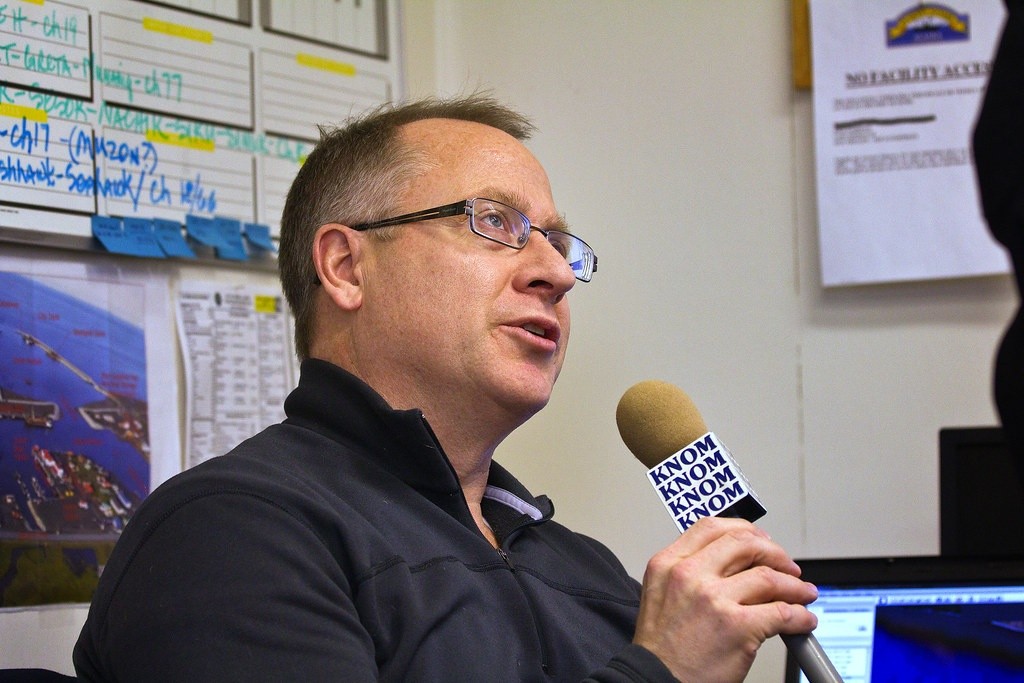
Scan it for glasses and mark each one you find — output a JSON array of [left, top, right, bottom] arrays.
[[315, 195, 599, 285]]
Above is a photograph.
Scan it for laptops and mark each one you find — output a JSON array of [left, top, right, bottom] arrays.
[[784, 583, 1024, 683]]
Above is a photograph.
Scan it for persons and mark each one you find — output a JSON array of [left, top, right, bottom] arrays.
[[71, 97, 819, 683]]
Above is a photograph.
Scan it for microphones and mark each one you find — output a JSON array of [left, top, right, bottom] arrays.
[[616, 380, 845, 683]]
